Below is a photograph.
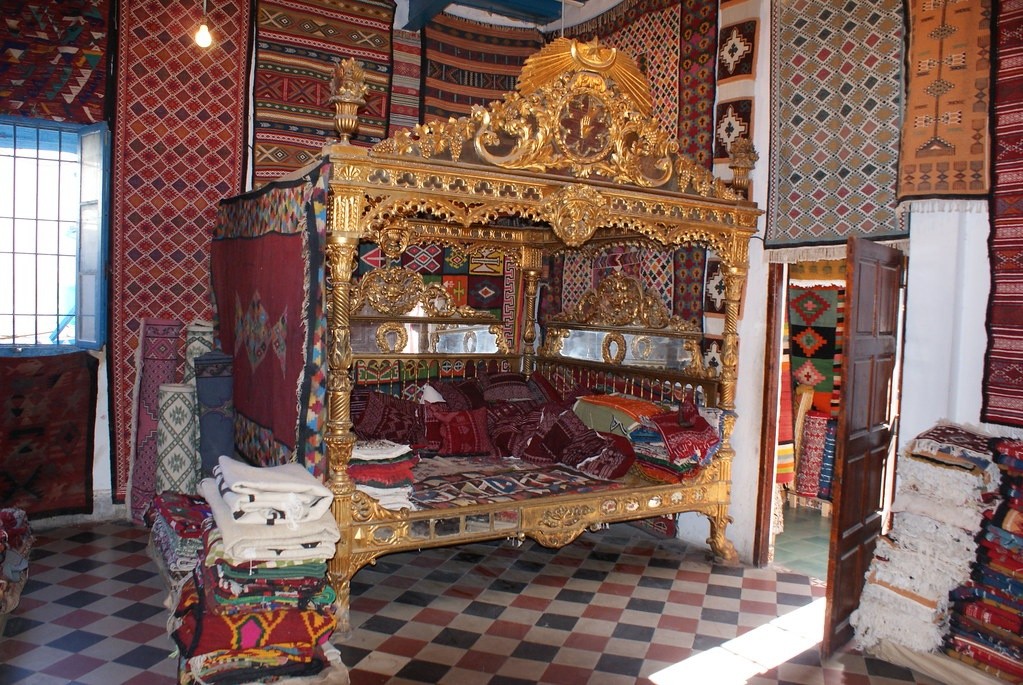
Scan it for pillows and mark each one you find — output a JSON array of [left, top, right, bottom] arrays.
[[527, 371, 563, 405], [352, 392, 420, 441], [431, 378, 487, 409], [476, 372, 533, 402], [422, 400, 461, 450], [350, 390, 422, 423], [431, 407, 497, 457]]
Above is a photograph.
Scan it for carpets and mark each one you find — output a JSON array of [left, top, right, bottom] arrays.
[[0, 1, 1023, 685]]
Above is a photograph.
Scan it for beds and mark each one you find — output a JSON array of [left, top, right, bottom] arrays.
[[283, 38, 766, 630]]
